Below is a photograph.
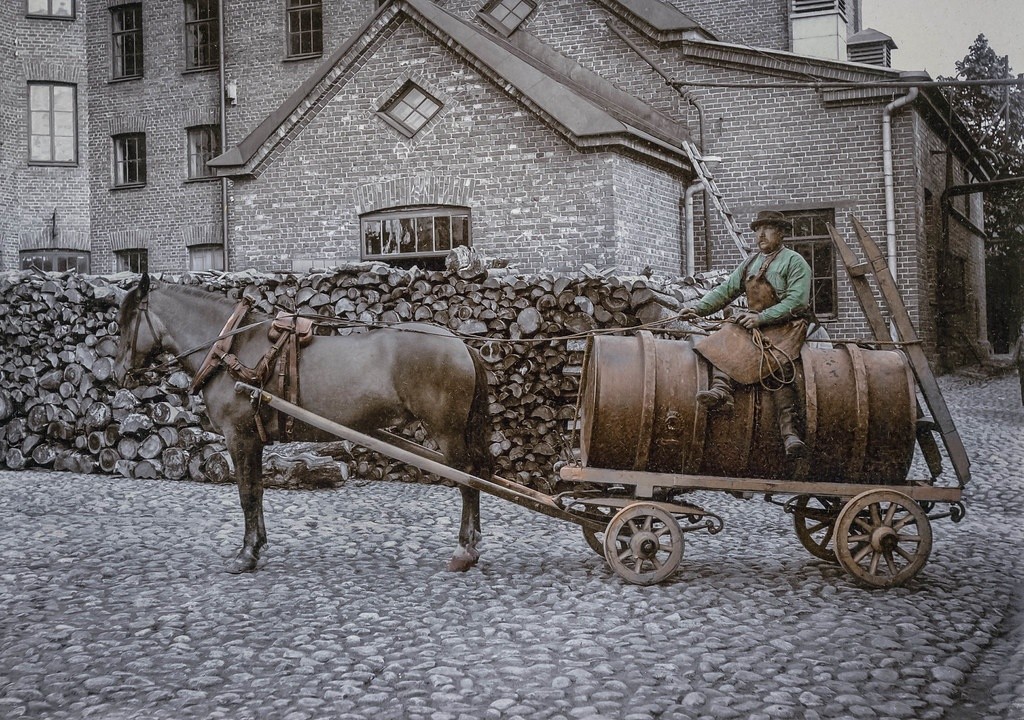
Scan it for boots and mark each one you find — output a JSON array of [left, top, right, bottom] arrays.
[[772, 386, 808, 456], [696, 365, 735, 416]]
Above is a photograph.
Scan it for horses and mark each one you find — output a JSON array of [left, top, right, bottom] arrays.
[[110, 272, 490, 574]]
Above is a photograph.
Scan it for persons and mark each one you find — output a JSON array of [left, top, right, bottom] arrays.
[[676, 210, 814, 460]]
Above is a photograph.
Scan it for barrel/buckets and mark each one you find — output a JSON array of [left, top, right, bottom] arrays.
[[580, 333, 917, 485]]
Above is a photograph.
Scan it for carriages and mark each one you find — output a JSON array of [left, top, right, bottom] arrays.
[[111, 258, 975, 588]]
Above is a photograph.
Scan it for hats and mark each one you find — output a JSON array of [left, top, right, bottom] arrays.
[[749, 210, 793, 233]]
